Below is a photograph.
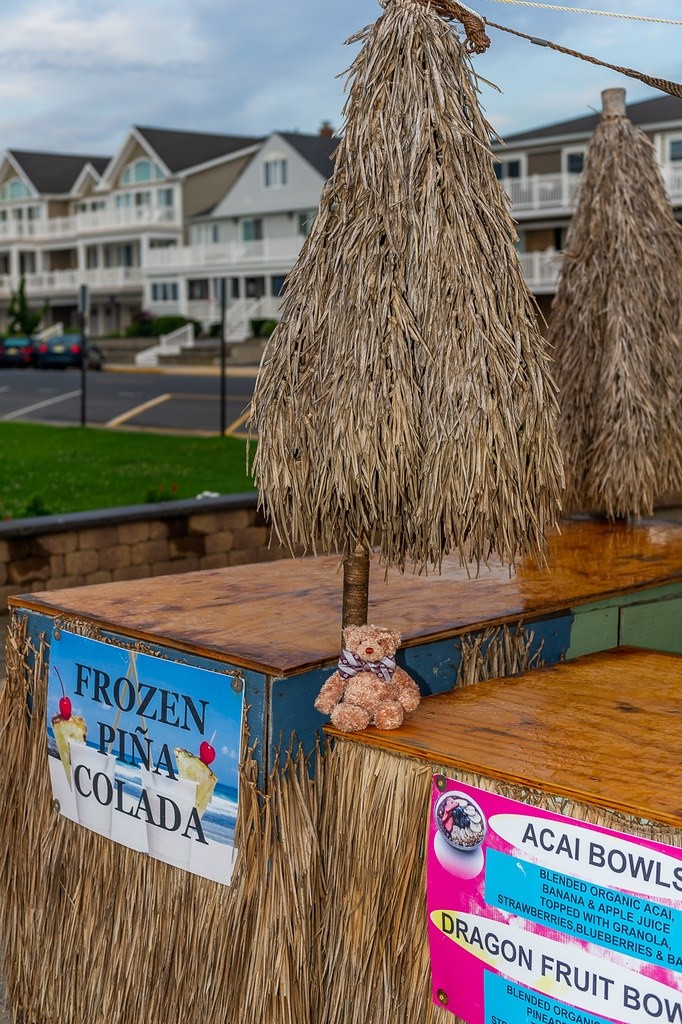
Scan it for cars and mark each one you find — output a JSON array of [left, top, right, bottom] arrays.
[[37, 334, 104, 374], [0, 337, 36, 369]]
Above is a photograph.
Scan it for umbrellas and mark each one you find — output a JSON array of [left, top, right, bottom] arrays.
[[542, 89, 681, 524], [246, 0, 567, 646]]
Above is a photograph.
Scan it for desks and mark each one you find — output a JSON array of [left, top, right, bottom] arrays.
[[7, 523, 682, 1024]]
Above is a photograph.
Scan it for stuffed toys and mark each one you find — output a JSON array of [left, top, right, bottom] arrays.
[[316, 624, 420, 733]]
